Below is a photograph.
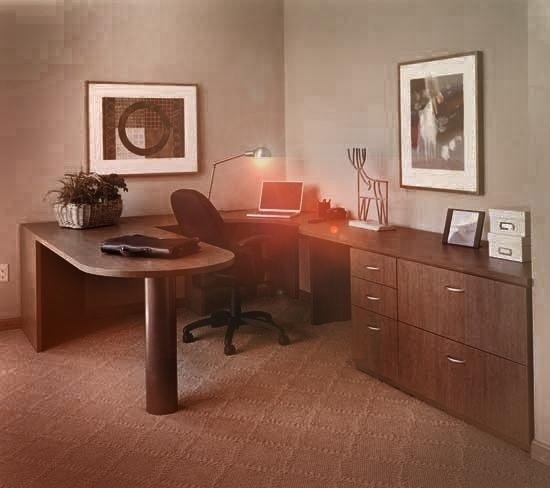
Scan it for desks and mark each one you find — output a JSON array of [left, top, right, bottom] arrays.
[[19, 209, 347, 415]]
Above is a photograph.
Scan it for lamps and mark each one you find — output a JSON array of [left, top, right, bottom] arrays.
[[208, 147, 273, 199]]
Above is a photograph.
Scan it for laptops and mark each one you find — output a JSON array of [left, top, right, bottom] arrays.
[[246, 180, 304, 218]]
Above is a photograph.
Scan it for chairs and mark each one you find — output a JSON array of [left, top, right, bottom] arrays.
[[170, 190, 290, 356]]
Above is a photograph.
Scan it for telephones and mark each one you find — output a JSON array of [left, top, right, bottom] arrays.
[[309, 207, 347, 223]]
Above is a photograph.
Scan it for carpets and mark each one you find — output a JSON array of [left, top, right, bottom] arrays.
[[0, 297, 550, 488]]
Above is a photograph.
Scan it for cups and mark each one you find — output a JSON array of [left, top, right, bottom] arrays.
[[318, 202, 330, 217]]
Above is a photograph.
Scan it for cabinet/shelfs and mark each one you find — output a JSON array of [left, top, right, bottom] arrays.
[[298, 227, 531, 453]]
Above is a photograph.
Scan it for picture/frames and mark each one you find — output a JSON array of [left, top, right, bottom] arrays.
[[397, 50, 483, 195], [85, 80, 200, 178]]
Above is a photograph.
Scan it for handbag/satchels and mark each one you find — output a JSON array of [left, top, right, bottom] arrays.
[[101, 235, 200, 258]]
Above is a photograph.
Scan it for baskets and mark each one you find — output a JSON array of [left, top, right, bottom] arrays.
[[54, 201, 122, 228]]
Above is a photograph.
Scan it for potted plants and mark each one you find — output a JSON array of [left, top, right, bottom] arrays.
[[46, 166, 128, 228]]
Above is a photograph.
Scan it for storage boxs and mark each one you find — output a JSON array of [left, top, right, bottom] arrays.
[[487, 232, 531, 262], [489, 205, 530, 237]]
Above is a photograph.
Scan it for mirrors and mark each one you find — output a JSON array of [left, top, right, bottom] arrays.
[[442, 208, 485, 249]]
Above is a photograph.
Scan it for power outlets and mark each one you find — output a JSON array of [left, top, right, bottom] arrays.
[[0, 263, 9, 283]]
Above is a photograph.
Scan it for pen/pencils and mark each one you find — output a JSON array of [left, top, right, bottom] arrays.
[[317, 199, 331, 208]]
